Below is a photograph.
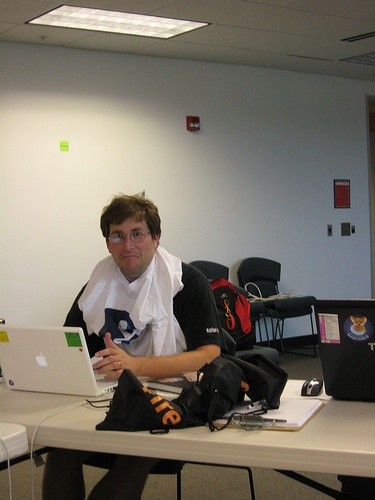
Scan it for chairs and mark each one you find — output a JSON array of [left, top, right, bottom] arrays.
[[235, 257, 318, 358], [188, 261, 280, 369]]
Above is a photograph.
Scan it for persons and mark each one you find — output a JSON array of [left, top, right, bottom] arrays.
[[40, 190, 227, 500]]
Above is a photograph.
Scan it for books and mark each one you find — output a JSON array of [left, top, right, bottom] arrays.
[[145, 373, 203, 394]]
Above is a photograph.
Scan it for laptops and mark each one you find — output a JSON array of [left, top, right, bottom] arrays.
[[314, 299, 375, 402], [0, 324, 151, 397]]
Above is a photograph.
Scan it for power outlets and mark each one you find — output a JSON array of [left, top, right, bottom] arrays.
[[341, 223, 351, 237]]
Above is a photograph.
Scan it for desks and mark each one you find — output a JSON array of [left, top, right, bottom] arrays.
[[1, 422, 30, 470], [1, 374, 375, 496]]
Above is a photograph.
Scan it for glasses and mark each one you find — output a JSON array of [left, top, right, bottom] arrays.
[[84, 395, 115, 410], [107, 229, 152, 245]]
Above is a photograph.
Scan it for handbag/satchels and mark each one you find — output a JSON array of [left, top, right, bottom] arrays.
[[97, 355, 289, 434], [244, 281, 301, 301]]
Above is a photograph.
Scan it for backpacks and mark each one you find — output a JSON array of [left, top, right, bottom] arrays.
[[210, 274, 257, 354]]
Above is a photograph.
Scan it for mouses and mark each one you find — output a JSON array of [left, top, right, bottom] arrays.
[[301, 377, 323, 396]]
